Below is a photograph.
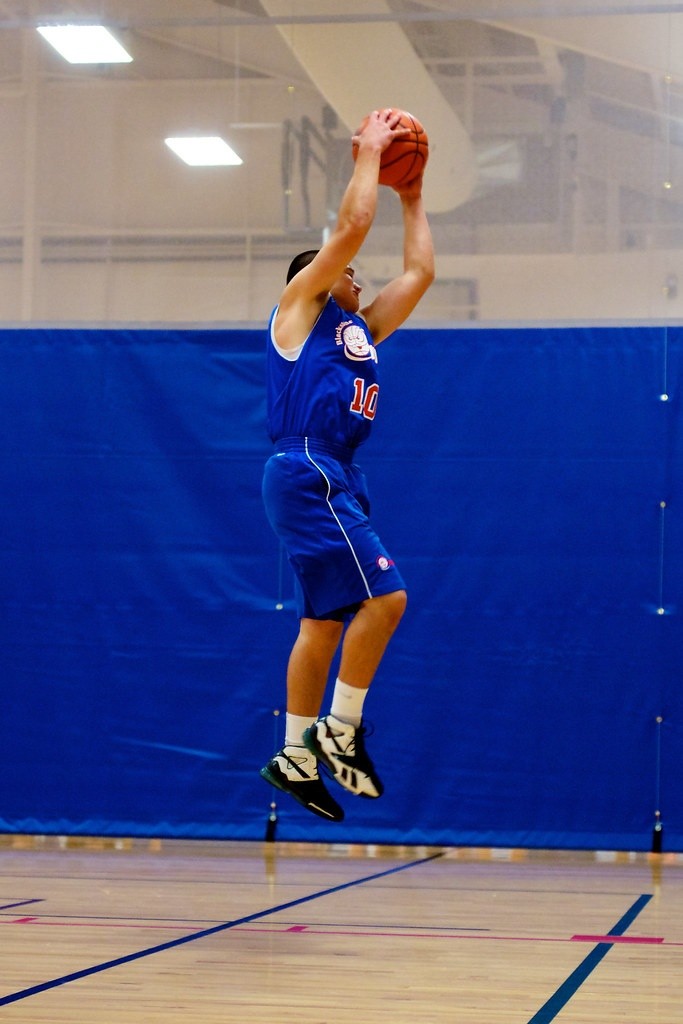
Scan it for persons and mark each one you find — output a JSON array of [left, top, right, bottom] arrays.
[[261, 108, 436, 823]]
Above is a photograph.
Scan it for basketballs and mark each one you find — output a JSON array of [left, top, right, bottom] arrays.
[[352, 107, 429, 187]]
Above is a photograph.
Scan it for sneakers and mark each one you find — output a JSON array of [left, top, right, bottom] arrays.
[[304, 713, 383, 800], [258, 743, 345, 822]]
